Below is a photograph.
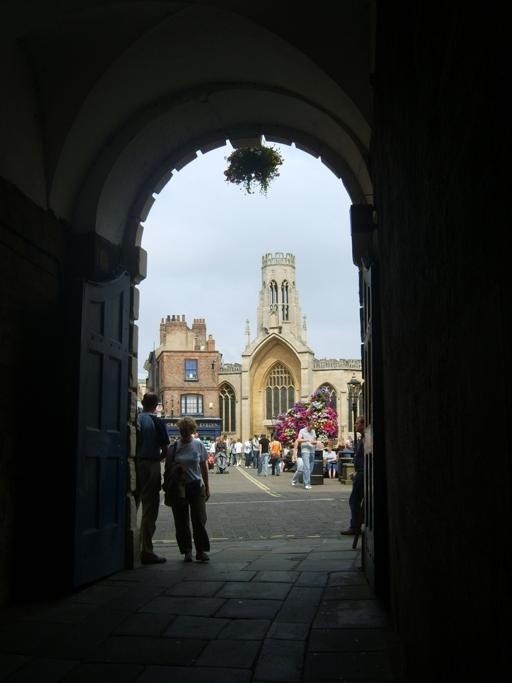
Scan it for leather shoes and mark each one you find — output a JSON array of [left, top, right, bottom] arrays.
[[341, 528, 361, 535]]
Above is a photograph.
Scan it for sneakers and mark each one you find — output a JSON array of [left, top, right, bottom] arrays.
[[140, 550, 167, 564], [184, 552, 193, 562], [195, 552, 210, 560], [304, 484, 312, 488], [292, 481, 297, 486]]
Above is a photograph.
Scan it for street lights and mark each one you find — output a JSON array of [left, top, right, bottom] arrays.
[[347, 372, 363, 460]]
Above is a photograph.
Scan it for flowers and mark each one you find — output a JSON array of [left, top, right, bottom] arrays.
[[274, 390, 338, 444]]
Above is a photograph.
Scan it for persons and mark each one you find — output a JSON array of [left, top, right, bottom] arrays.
[[195, 421, 340, 490], [340, 415, 366, 535], [136, 391, 172, 565], [164, 415, 212, 563]]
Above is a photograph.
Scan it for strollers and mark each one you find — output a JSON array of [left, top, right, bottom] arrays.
[[215, 451, 232, 473]]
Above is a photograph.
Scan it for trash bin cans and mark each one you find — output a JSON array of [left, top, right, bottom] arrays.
[[310, 450, 324, 485]]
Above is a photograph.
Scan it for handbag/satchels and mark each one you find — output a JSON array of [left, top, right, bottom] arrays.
[[170, 465, 202, 507], [268, 453, 279, 464]]
